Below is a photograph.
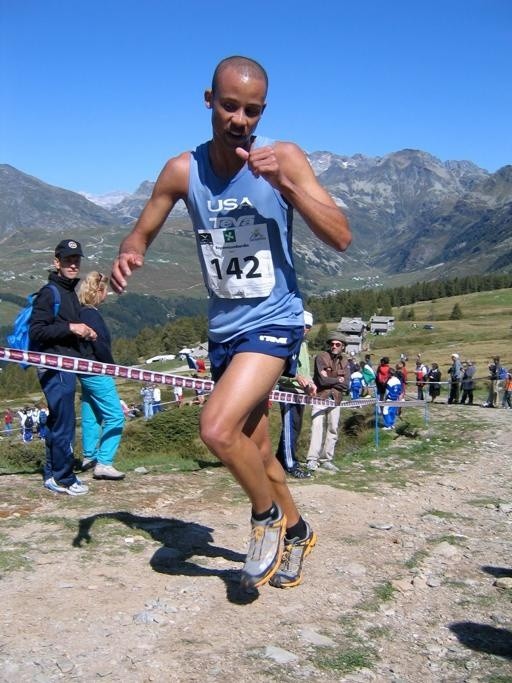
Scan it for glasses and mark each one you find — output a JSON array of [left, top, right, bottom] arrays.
[[305, 322, 311, 330], [328, 343, 344, 347]]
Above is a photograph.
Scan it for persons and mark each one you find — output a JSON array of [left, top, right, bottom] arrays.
[[4, 407, 50, 444], [152, 384, 162, 415], [348, 352, 512, 431], [306, 332, 350, 471], [196, 389, 205, 407], [108, 55, 353, 595], [140, 384, 153, 420], [76, 271, 126, 480], [173, 385, 183, 408], [275, 309, 318, 479], [29, 239, 98, 496]]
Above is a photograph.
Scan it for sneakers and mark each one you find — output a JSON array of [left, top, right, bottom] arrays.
[[306, 460, 337, 472], [287, 467, 311, 479], [82, 456, 126, 482], [42, 476, 88, 496], [237, 507, 318, 592], [381, 426, 396, 431]]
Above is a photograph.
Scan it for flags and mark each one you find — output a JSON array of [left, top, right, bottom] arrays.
[[186, 354, 206, 371]]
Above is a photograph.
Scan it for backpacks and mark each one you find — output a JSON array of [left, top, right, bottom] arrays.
[[24, 416, 33, 428], [39, 412, 48, 425], [6, 285, 62, 370]]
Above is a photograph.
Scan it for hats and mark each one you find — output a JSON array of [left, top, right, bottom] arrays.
[[303, 309, 314, 327], [326, 331, 349, 346], [54, 239, 85, 258]]
